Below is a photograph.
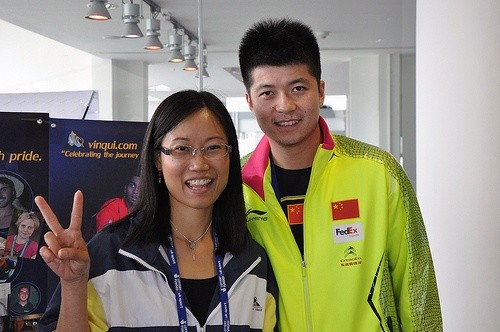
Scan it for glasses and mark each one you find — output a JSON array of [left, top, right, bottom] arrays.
[[159, 144, 233, 161]]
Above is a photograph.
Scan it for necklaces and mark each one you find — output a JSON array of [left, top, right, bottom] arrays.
[[170, 220, 212, 249]]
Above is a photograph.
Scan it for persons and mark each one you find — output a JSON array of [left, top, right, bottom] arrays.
[[96, 175, 142, 231], [239, 20, 443, 332], [0, 241, 15, 281], [5, 212, 39, 260], [35, 90, 279, 332], [0, 175, 24, 228], [11, 286, 32, 312]]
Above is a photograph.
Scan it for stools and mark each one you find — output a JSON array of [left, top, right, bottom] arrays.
[[9, 313, 43, 332]]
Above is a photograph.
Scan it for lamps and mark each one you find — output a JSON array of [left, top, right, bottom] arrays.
[[143, 6, 164, 50], [120, 0, 144, 39], [195, 44, 210, 78], [83, 0, 112, 21], [21, 117, 57, 128], [167, 23, 186, 63], [181, 35, 199, 71]]
